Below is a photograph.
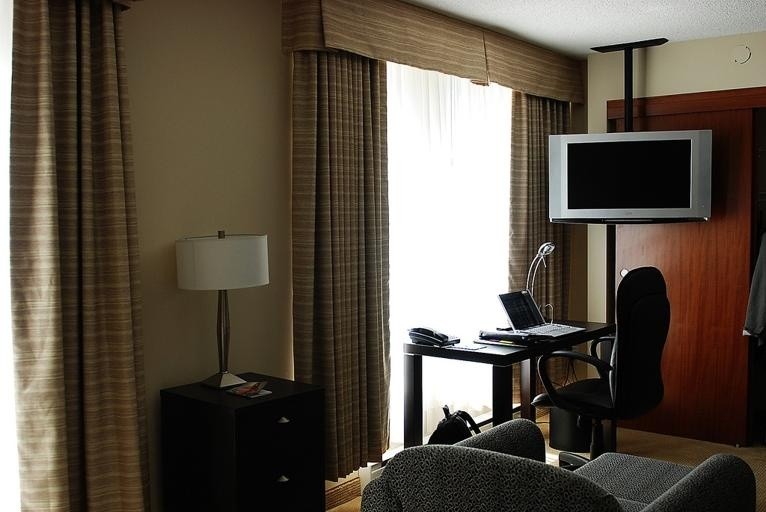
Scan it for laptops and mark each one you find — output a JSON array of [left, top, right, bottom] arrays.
[[498, 289, 586, 338]]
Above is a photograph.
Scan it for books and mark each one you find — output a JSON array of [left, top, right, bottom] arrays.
[[447, 341, 486, 352], [226, 380, 271, 402]]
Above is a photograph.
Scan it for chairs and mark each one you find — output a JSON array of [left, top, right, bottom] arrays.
[[531, 263, 668, 469]]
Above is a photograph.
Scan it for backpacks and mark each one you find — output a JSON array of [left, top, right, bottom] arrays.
[[426, 403, 480, 444]]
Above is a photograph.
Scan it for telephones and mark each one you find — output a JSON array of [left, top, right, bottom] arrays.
[[409, 328, 460, 345]]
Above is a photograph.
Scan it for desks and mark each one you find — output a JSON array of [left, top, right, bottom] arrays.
[[401, 315, 615, 445]]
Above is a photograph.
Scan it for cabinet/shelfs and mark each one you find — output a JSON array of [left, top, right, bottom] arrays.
[[155, 372, 328, 512], [598, 86, 765, 450]]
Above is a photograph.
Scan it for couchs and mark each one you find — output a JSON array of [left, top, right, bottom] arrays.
[[358, 419, 757, 511]]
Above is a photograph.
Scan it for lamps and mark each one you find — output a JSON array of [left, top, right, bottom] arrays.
[[168, 224, 272, 388]]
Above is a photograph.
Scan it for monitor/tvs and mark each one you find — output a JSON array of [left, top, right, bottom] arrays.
[[547, 129, 713, 224]]
